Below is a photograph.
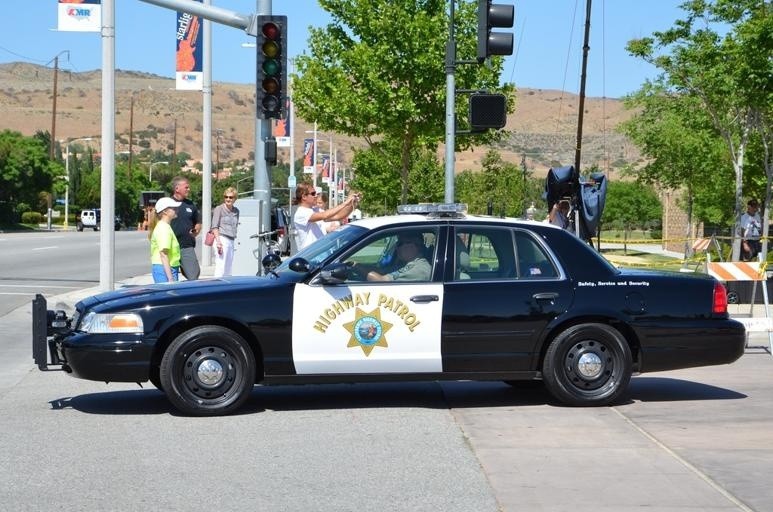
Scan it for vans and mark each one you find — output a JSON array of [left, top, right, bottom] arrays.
[[74, 209, 122, 232], [270, 198, 290, 257]]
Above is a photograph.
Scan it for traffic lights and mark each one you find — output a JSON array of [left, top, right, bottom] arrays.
[[476, 0, 514, 61], [469, 94, 506, 128], [256, 15, 286, 120]]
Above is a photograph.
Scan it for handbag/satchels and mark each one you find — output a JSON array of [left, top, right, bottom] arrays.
[[206, 231, 215, 245]]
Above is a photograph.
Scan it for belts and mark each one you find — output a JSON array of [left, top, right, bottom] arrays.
[[220, 234, 234, 240]]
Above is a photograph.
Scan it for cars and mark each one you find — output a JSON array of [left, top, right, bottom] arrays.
[[30, 202, 745, 418]]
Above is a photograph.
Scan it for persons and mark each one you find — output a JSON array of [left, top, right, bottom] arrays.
[[167, 175, 201, 280], [313, 192, 340, 234], [347, 231, 432, 283], [291, 182, 362, 252], [146, 197, 182, 282], [740, 199, 762, 262], [203, 186, 239, 277], [548, 200, 571, 230]]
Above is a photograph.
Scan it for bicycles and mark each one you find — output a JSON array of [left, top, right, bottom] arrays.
[[251, 226, 283, 275]]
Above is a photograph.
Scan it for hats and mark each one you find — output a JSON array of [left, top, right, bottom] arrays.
[[155, 197, 182, 213], [747, 200, 759, 207]]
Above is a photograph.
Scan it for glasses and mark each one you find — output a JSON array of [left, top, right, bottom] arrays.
[[396, 240, 413, 246], [305, 192, 316, 196], [225, 196, 233, 199]]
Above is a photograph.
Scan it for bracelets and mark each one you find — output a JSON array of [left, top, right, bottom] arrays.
[[743, 240, 748, 243]]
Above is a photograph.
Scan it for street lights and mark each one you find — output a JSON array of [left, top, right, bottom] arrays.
[[242, 43, 297, 204], [303, 130, 346, 209], [148, 161, 168, 183], [114, 151, 131, 156], [62, 137, 93, 232]]
